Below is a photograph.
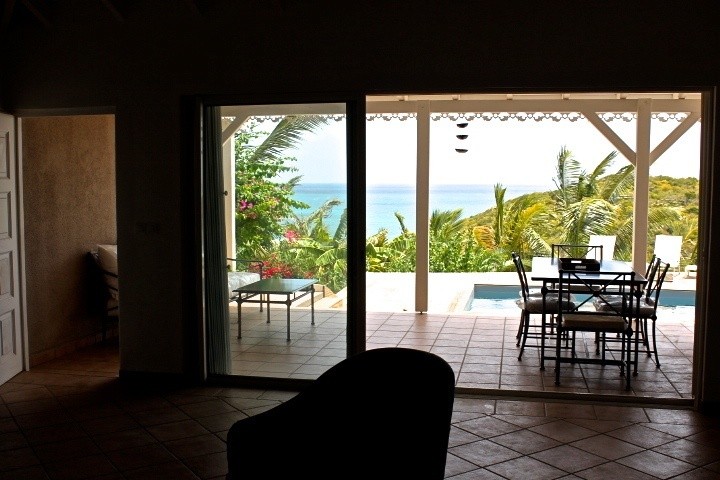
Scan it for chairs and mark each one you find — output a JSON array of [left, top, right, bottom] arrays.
[[512, 255, 577, 363], [511, 252, 572, 345], [555, 269, 635, 389], [551, 243, 603, 335], [226, 347, 455, 480], [593, 255, 659, 343], [586, 235, 618, 259], [597, 258, 671, 367], [653, 234, 685, 278]]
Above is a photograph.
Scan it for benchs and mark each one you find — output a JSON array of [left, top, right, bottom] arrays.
[[81, 245, 264, 340]]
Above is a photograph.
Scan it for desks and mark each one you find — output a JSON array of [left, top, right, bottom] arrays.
[[532, 256, 648, 376], [231, 278, 318, 343]]
